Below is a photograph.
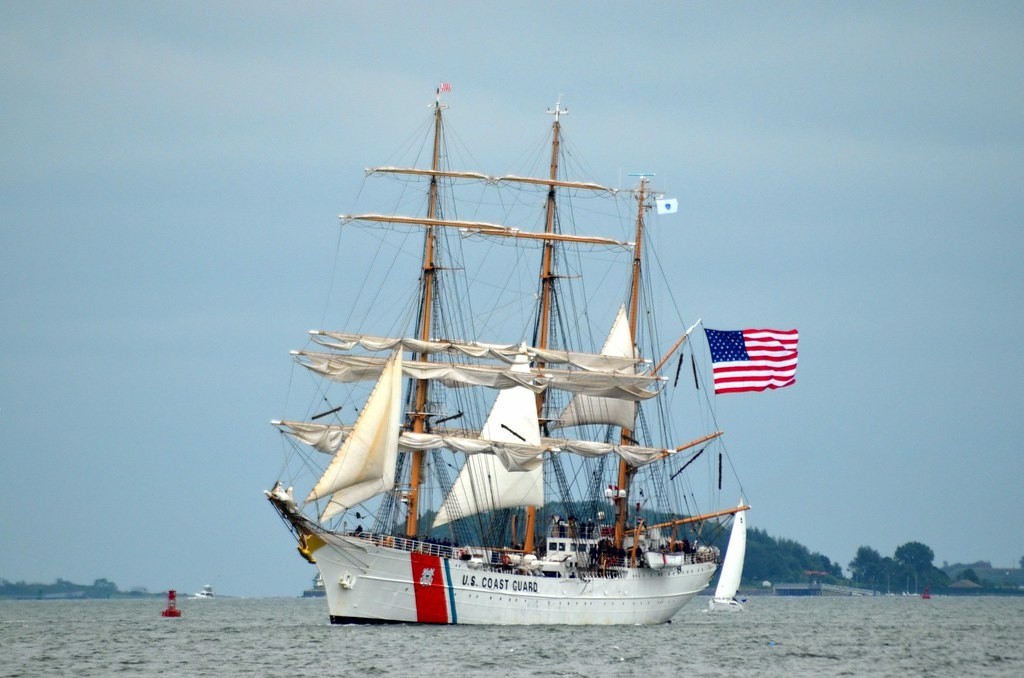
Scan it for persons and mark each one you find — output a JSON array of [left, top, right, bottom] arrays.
[[590, 544, 597, 564], [659, 537, 696, 553], [587, 519, 595, 539], [397, 533, 451, 556], [354, 525, 363, 536], [635, 545, 642, 558], [580, 521, 586, 539], [557, 516, 565, 537]]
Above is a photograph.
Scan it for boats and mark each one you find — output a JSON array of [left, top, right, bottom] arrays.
[[188, 582, 216, 603], [921, 584, 929, 599]]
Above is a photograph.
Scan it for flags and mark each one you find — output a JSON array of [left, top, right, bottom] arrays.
[[441, 83, 451, 93], [704, 327, 799, 394], [657, 199, 678, 214]]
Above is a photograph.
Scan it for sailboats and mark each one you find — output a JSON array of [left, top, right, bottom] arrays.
[[303, 574, 325, 598], [261, 80, 752, 628], [706, 498, 748, 611]]
